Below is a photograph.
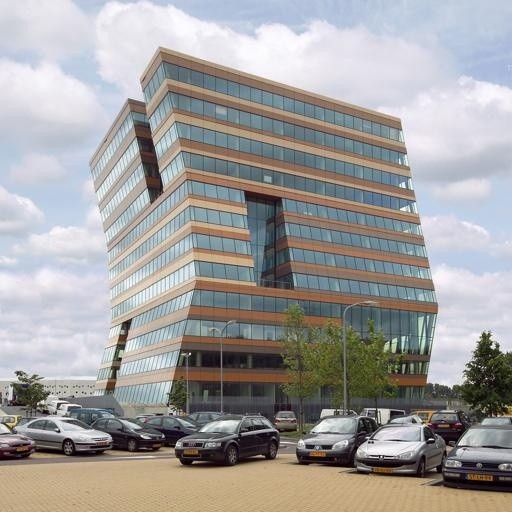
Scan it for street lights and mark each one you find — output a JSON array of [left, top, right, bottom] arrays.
[[209, 319, 238, 416], [181, 352, 191, 415], [343, 301, 381, 416]]
